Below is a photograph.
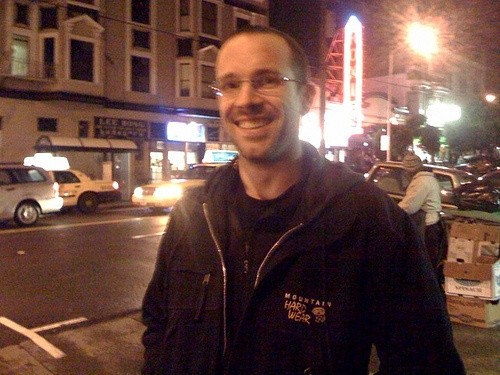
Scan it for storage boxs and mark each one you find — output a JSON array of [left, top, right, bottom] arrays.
[[442, 223, 500, 328]]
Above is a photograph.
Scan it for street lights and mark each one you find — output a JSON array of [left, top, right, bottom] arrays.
[[385, 23, 440, 162]]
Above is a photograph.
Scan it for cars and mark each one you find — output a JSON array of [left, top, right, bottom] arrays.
[[131, 162, 228, 215], [364, 152, 500, 212], [47, 170, 122, 214]]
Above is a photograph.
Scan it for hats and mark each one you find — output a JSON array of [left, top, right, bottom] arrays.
[[403, 152, 422, 171]]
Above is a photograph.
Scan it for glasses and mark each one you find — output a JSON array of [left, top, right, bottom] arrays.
[[208, 75, 301, 98]]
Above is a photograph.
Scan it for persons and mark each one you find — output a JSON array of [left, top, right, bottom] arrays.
[[397, 154, 449, 285], [136, 23, 466, 375]]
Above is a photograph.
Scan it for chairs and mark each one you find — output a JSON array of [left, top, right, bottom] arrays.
[[378, 178, 400, 192]]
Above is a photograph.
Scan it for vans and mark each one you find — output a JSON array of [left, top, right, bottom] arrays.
[[0, 162, 65, 228]]
